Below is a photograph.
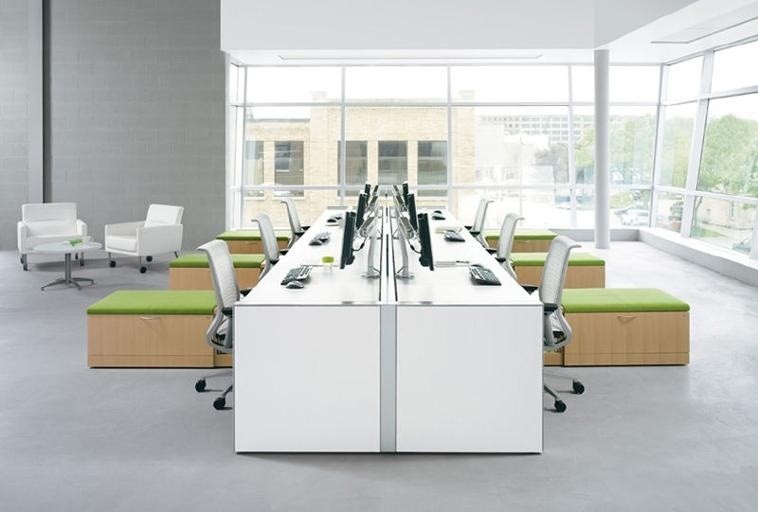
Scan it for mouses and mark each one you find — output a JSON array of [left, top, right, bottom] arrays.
[[286, 281, 304, 289], [447, 229, 454, 231], [470, 264, 483, 267], [327, 218, 336, 222], [309, 240, 323, 245], [434, 210, 442, 214]]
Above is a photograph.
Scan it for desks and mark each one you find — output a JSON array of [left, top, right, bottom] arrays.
[[234, 205, 544, 455], [34, 242, 102, 291]]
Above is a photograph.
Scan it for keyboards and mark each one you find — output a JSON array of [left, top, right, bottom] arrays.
[[313, 232, 331, 240], [334, 215, 343, 219], [443, 231, 465, 242], [469, 267, 500, 285], [281, 266, 313, 284], [432, 214, 445, 219]]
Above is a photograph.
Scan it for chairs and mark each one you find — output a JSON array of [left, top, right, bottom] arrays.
[[17, 202, 87, 270], [105, 203, 184, 273]]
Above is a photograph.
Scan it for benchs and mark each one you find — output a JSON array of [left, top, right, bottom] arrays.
[[509, 252, 606, 288], [86, 290, 242, 368], [543, 288, 691, 365], [169, 254, 266, 291]]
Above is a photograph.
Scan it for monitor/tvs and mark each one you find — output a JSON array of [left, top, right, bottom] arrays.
[[340, 212, 356, 269], [406, 193, 417, 233], [355, 193, 367, 230], [418, 213, 434, 271], [402, 183, 408, 206], [365, 184, 371, 198]]
